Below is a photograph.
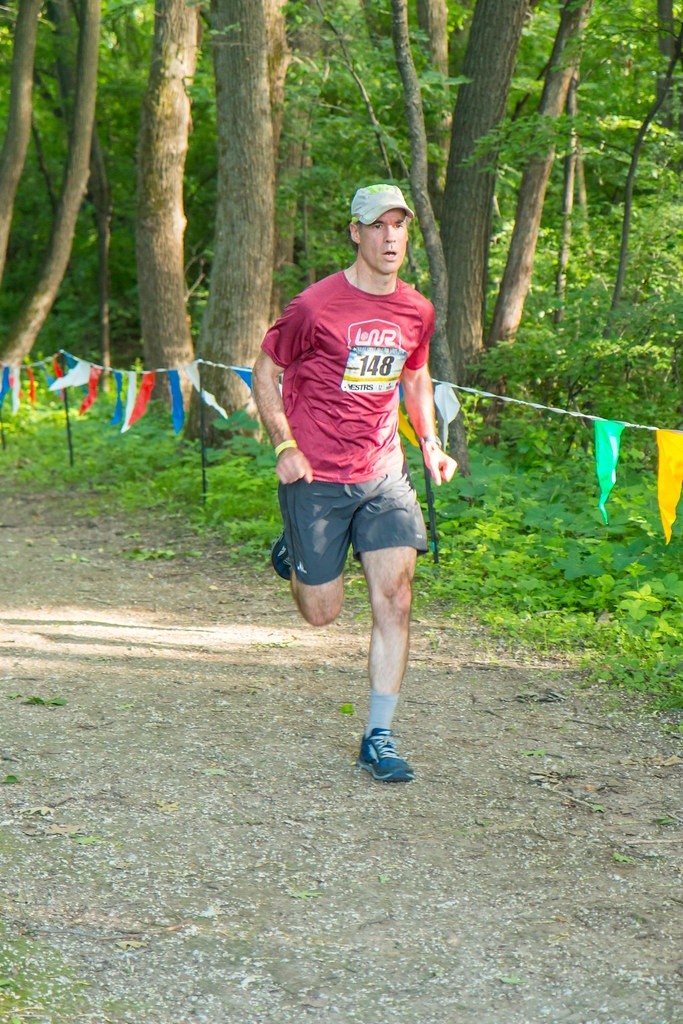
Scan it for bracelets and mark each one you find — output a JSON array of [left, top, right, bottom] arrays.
[[421, 436, 443, 446], [274, 440, 298, 456]]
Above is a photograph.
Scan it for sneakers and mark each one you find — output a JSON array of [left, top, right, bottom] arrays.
[[356, 727, 415, 782], [272, 530, 291, 581]]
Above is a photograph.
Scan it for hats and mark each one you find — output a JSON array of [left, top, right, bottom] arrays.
[[351, 184, 414, 226]]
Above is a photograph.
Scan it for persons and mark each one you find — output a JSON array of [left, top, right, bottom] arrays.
[[253, 183, 458, 782]]
